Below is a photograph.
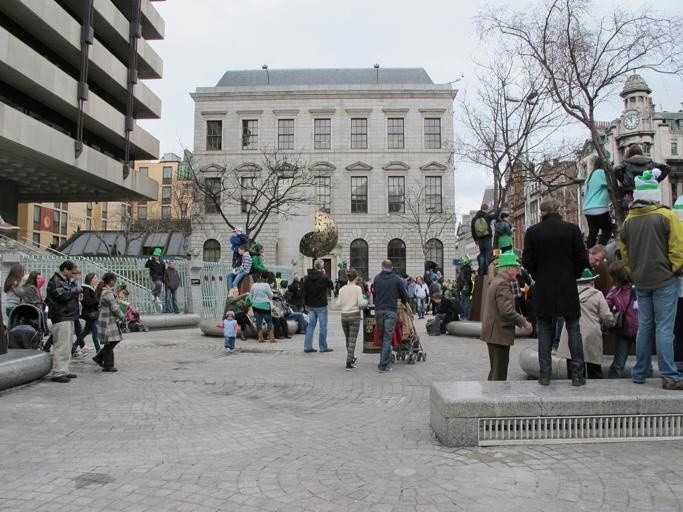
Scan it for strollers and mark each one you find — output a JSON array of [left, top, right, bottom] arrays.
[[390, 298, 428, 365], [5, 298, 47, 351], [118, 303, 148, 333]]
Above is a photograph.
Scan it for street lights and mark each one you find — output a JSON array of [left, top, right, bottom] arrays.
[[372, 61, 380, 83], [261, 63, 269, 84], [501, 91, 540, 228]]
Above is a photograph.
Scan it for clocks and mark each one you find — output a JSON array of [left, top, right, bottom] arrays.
[[623, 114, 639, 130]]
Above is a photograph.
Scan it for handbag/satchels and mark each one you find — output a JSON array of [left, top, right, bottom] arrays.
[[271, 308, 282, 319], [498, 235, 513, 248], [245, 294, 251, 305], [252, 300, 270, 310], [601, 310, 625, 331]]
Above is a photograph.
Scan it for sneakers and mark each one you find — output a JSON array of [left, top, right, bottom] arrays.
[[304, 348, 333, 352], [277, 336, 291, 338], [51, 374, 77, 382], [378, 367, 392, 372], [225, 348, 234, 352], [93, 357, 118, 372], [662, 379, 683, 390], [298, 329, 305, 333], [539, 376, 549, 385], [428, 331, 440, 336], [346, 356, 357, 371], [73, 345, 95, 359], [152, 295, 160, 302], [572, 377, 585, 385]]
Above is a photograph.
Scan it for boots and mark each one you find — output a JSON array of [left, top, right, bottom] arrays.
[[241, 324, 277, 343]]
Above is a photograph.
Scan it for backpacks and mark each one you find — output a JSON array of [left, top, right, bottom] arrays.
[[475, 218, 491, 237]]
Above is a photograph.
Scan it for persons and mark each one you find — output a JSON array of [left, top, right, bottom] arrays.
[[357, 278, 374, 297], [144, 248, 166, 303], [470, 202, 500, 277], [604, 260, 654, 379], [371, 257, 409, 373], [400, 263, 474, 332], [521, 198, 589, 386], [480, 252, 534, 381], [583, 245, 606, 265], [612, 144, 672, 216], [214, 310, 239, 354], [301, 258, 335, 353], [335, 268, 369, 371], [91, 270, 127, 372], [511, 268, 533, 319], [557, 267, 614, 379], [582, 157, 613, 245], [226, 226, 310, 343], [44, 260, 85, 384], [1, 264, 134, 354], [163, 258, 182, 314], [615, 166, 683, 391], [491, 212, 514, 260]]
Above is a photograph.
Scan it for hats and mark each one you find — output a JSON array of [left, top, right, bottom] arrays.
[[576, 268, 600, 282], [153, 248, 161, 256], [496, 254, 521, 268], [226, 311, 235, 317], [633, 168, 662, 201], [673, 196, 683, 217], [462, 254, 473, 266], [232, 227, 241, 232], [255, 243, 263, 252]]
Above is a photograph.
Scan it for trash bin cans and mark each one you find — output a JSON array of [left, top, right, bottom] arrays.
[[363, 304, 381, 353]]
